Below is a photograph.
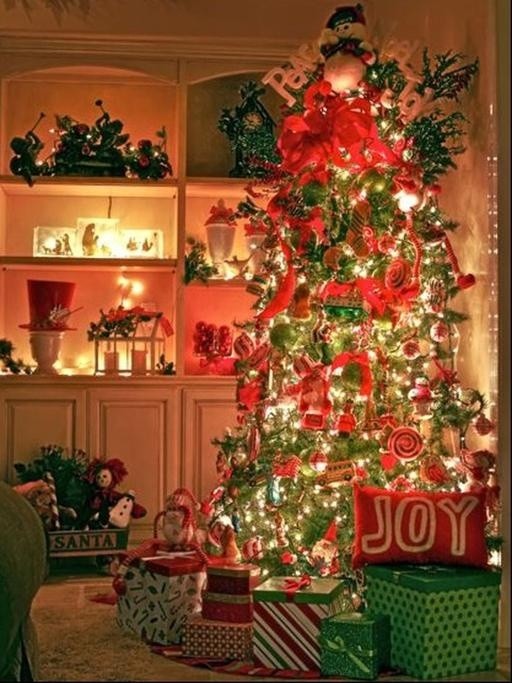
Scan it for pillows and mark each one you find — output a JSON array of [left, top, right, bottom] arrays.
[[344, 477, 487, 573]]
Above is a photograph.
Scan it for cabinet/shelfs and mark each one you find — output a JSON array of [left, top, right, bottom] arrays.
[[1, 29, 323, 565]]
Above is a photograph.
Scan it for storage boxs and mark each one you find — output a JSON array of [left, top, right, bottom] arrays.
[[317, 612, 393, 681], [200, 589, 254, 623], [182, 612, 252, 661], [249, 573, 346, 672], [364, 563, 499, 680], [203, 566, 260, 592], [114, 550, 230, 645]]
